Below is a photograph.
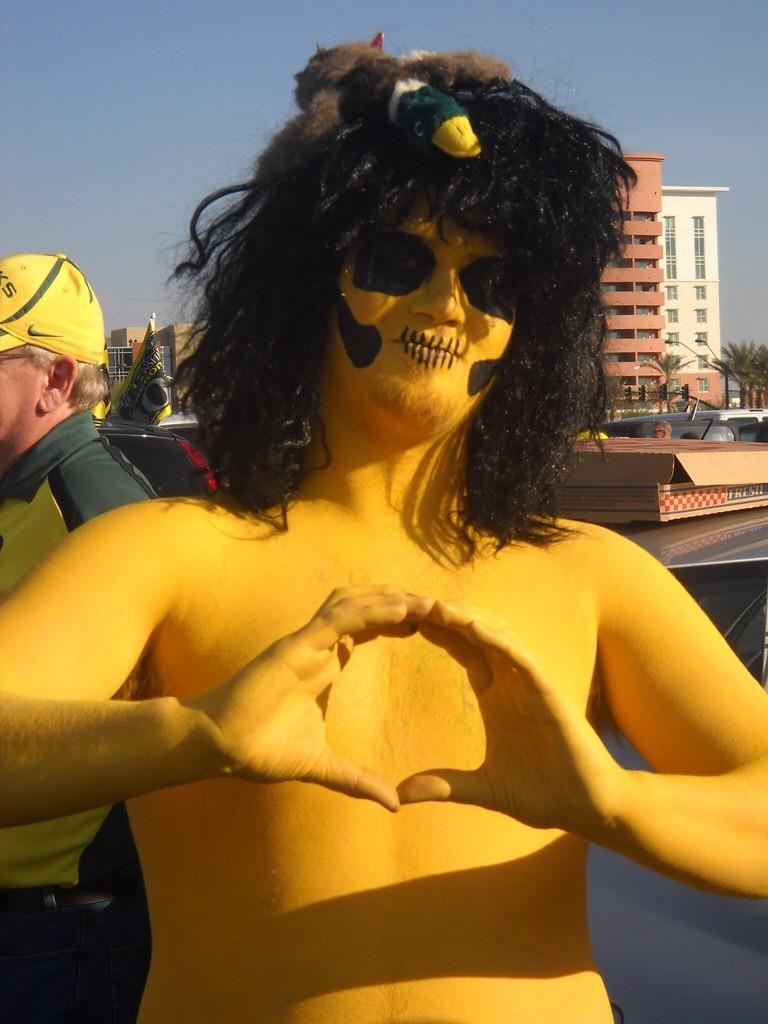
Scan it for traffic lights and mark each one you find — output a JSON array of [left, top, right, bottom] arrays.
[[624, 387, 631, 403], [638, 385, 645, 401], [659, 384, 666, 400], [681, 384, 689, 401]]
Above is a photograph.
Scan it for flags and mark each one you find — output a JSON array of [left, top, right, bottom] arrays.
[[105, 318, 171, 425]]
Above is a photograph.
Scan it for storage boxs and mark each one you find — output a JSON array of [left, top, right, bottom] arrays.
[[550, 437, 768, 524]]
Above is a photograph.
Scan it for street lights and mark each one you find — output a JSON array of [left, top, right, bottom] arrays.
[[665, 337, 729, 409]]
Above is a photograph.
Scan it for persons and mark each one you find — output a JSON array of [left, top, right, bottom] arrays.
[[0, 253, 165, 1024], [651, 419, 672, 439], [0, 38, 768, 1024], [679, 431, 700, 440]]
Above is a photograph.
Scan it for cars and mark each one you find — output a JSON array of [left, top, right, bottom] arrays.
[[157, 411, 205, 450], [629, 407, 768, 443], [91, 415, 214, 499], [597, 419, 741, 444]]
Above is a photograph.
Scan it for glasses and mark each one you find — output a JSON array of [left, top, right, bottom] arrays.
[[0, 350, 53, 361]]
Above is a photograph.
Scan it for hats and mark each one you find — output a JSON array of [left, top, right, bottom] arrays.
[[1, 251, 108, 367]]
